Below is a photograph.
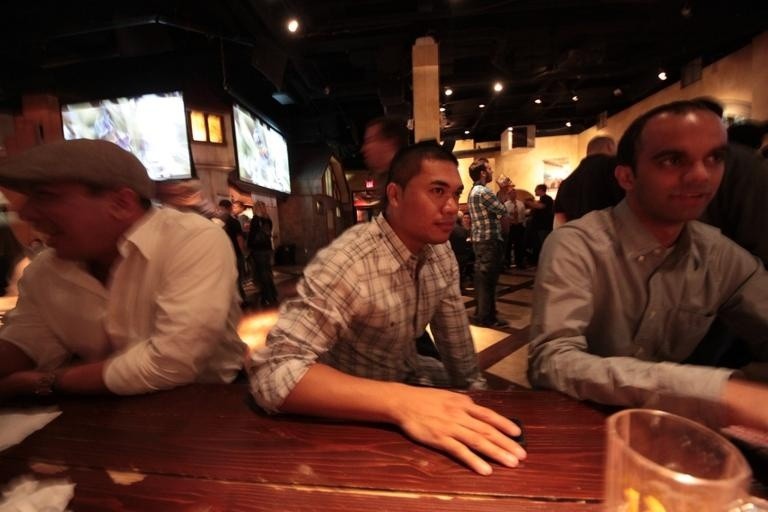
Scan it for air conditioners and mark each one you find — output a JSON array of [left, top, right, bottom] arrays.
[[500, 125, 536, 156]]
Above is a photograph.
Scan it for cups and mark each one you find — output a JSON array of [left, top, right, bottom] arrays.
[[497, 173, 514, 193], [603, 409, 768, 512]]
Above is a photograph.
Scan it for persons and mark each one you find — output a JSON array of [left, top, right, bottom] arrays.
[[244, 137, 527, 477], [215, 200, 277, 306], [449, 99, 767, 433], [1, 137, 247, 405], [360, 114, 404, 173]]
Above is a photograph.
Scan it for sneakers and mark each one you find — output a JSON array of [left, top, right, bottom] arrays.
[[474, 318, 510, 329]]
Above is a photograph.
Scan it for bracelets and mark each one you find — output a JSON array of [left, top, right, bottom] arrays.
[[33, 368, 57, 405]]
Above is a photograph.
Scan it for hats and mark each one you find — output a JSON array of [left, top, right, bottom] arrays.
[[0, 138, 152, 200]]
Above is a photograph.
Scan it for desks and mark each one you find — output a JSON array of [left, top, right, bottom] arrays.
[[0, 362, 768, 511]]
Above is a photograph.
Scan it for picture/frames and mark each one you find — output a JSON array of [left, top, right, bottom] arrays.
[[207, 113, 226, 145], [187, 111, 207, 143]]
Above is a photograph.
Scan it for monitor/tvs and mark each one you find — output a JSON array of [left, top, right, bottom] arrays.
[[60, 89, 199, 181], [231, 102, 296, 198]]
